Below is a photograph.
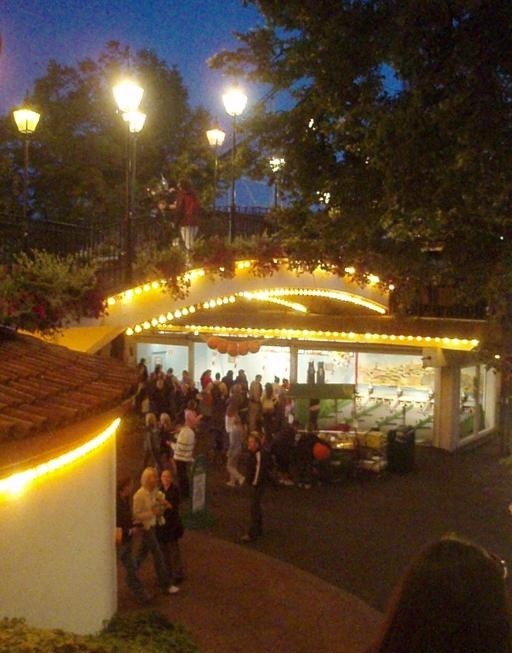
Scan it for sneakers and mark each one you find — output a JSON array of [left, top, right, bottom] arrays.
[[241, 534, 257, 541], [135, 584, 179, 605]]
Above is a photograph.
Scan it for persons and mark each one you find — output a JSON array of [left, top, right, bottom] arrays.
[[133, 359, 332, 504], [171, 178, 201, 253], [116, 466, 185, 601], [371, 536, 512, 651], [239, 430, 271, 542]]
